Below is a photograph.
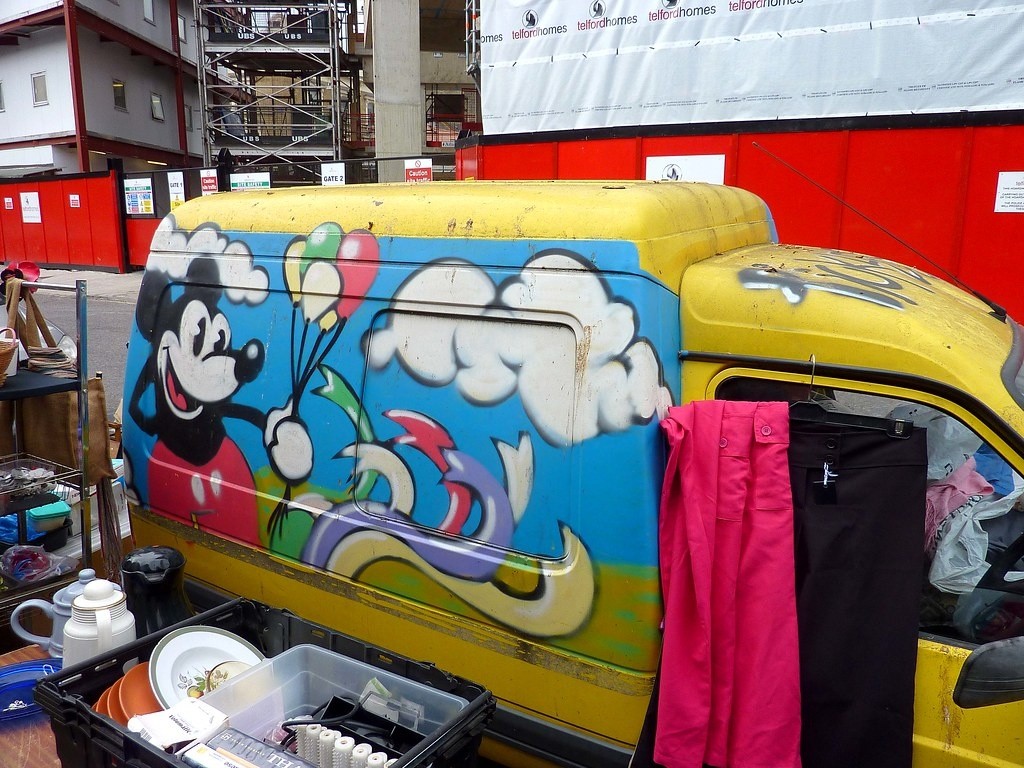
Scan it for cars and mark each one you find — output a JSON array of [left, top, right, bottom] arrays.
[[0, 292, 78, 394]]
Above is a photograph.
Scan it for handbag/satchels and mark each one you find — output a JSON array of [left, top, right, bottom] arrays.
[[5, 278, 78, 378], [22, 378, 118, 486]]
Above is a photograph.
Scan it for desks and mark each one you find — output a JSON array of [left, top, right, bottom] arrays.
[[0, 644, 63, 768]]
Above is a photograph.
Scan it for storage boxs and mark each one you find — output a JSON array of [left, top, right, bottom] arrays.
[[31, 596, 498, 768]]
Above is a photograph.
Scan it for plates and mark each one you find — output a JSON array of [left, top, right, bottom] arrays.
[[91, 626, 265, 727], [0, 657, 63, 723]]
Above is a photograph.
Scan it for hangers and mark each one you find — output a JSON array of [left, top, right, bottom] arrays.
[[787, 353, 914, 438]]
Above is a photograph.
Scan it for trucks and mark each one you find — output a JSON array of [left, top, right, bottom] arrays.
[[121, 180, 1023, 768]]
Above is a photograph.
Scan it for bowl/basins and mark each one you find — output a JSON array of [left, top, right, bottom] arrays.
[[27, 500, 71, 532], [206, 660, 253, 692]]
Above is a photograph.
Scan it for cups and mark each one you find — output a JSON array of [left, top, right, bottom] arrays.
[[63, 579, 136, 682], [9, 568, 121, 660]]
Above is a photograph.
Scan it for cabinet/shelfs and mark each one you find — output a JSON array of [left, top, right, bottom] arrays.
[[0, 276, 95, 627]]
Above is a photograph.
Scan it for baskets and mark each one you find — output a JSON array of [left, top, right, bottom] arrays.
[[0, 327, 18, 387], [33, 596, 497, 768]]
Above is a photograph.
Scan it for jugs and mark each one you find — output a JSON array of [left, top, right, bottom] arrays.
[[120, 544, 197, 637]]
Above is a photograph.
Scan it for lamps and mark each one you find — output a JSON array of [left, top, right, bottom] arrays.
[[457, 129, 473, 140]]
